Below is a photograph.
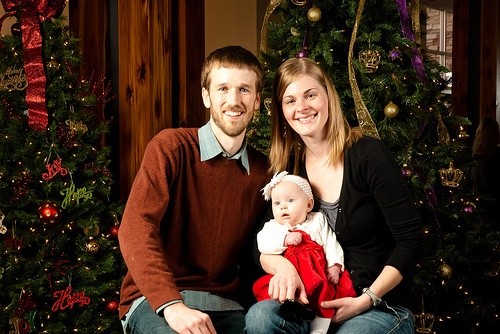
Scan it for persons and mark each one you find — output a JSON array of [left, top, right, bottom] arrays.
[[117, 45, 275, 334], [252, 171, 356, 334], [242, 56, 442, 334]]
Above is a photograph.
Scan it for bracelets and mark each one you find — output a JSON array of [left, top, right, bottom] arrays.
[[361, 287, 382, 307]]
